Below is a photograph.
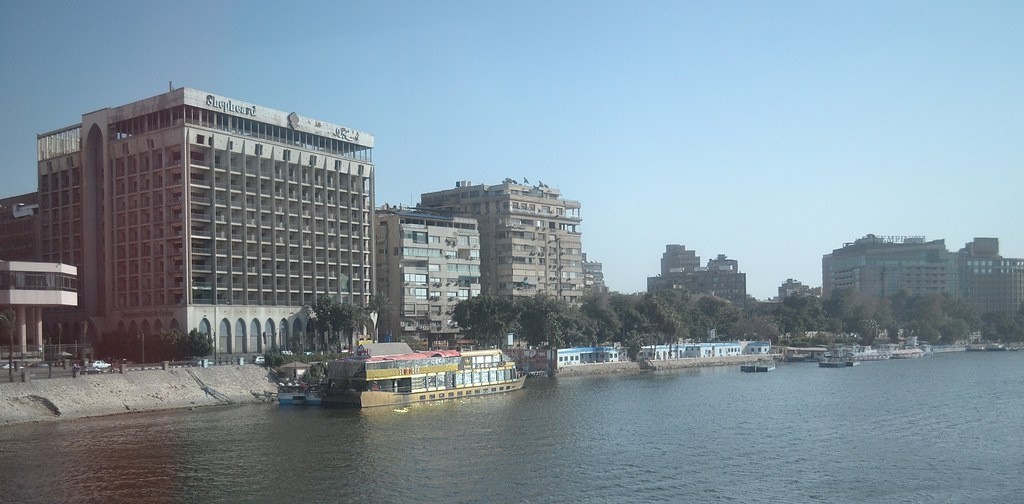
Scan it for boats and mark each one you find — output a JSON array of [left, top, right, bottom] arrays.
[[277, 384, 323, 406], [321, 342, 529, 409], [818, 350, 857, 368]]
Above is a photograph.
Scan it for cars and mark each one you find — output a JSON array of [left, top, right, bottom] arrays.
[[1, 361, 26, 370], [249, 355, 266, 365], [30, 362, 49, 368], [90, 360, 112, 367], [80, 364, 103, 374]]
